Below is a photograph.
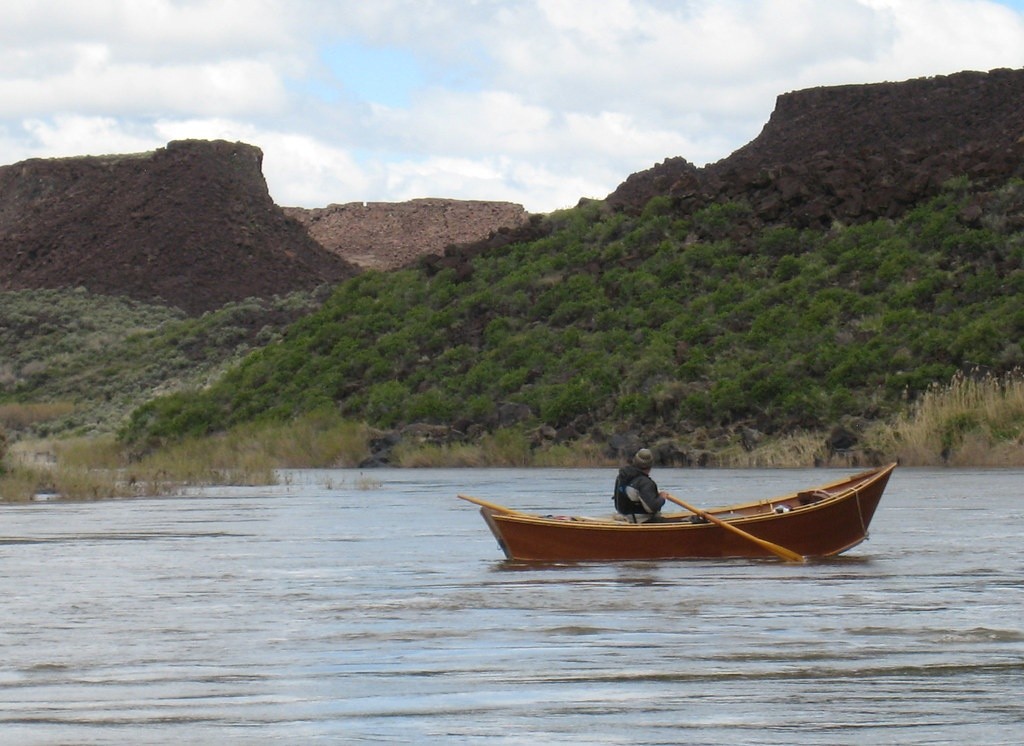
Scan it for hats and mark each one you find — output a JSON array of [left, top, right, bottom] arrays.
[[634, 449, 655, 469]]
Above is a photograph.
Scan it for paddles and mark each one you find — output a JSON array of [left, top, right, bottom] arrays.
[[664, 493, 804, 562], [456, 493, 516, 513]]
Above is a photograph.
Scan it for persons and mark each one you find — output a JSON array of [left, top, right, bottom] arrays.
[[611, 448, 689, 523]]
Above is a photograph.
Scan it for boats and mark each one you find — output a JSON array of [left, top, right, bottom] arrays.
[[457, 460, 898, 562]]
